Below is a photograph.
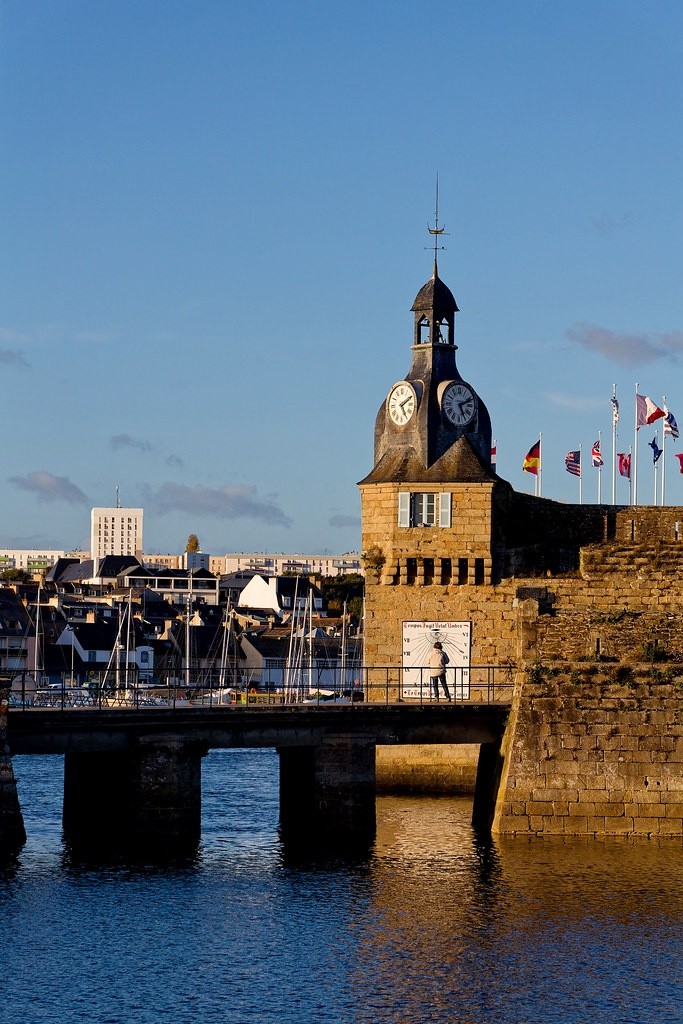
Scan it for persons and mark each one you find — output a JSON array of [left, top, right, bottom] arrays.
[[427, 642, 451, 703], [251, 688, 257, 694]]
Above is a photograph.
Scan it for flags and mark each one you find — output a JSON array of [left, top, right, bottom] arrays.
[[617, 454, 631, 478], [648, 437, 663, 465], [609, 396, 619, 424], [663, 405, 679, 441], [522, 440, 541, 475], [592, 441, 604, 467], [491, 446, 496, 463], [636, 394, 666, 425], [675, 454, 683, 474], [565, 451, 580, 477]]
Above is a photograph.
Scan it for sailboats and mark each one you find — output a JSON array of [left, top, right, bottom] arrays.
[[0, 575, 364, 706]]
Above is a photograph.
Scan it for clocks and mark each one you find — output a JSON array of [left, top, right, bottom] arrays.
[[389, 385, 414, 425], [443, 385, 475, 425]]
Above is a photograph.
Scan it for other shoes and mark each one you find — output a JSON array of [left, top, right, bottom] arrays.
[[446, 698, 451, 702], [433, 698, 439, 702]]
